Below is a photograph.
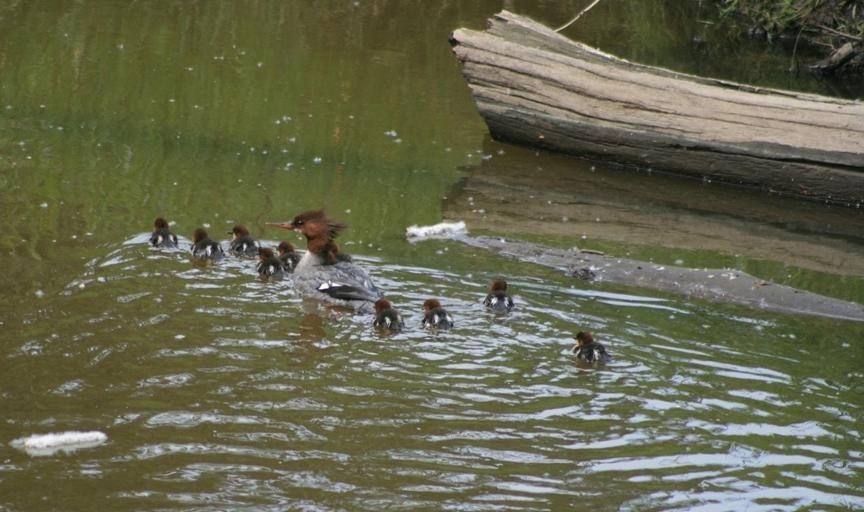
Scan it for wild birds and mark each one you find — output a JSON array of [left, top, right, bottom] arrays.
[[556, 261, 595, 281], [419, 299, 455, 330], [373, 299, 405, 331], [482, 278, 515, 315], [226, 224, 261, 258], [148, 217, 179, 249], [255, 244, 284, 282], [263, 208, 386, 314], [570, 331, 612, 365], [190, 228, 226, 261], [275, 240, 304, 271]]
